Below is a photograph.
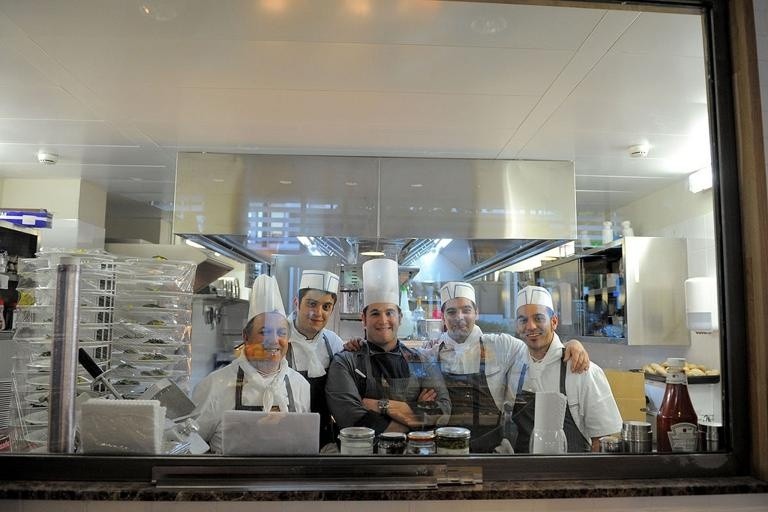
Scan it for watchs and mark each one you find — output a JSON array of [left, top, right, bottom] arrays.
[[376, 394, 391, 414]]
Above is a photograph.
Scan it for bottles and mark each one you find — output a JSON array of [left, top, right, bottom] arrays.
[[405, 430, 436, 458], [397, 285, 426, 339], [0, 298, 17, 331], [217, 277, 240, 299], [620, 420, 652, 454], [597, 436, 623, 453], [697, 421, 726, 454], [432, 304, 439, 319], [578, 219, 634, 253], [335, 426, 375, 457], [376, 432, 407, 456], [653, 357, 700, 454], [0, 249, 18, 275], [434, 426, 471, 457]]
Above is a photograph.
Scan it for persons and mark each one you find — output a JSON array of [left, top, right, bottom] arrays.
[[324, 299, 453, 450], [188, 308, 313, 458], [499, 285, 624, 454], [281, 269, 346, 452], [340, 282, 591, 453]]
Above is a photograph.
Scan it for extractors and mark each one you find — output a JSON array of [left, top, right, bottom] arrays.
[[463, 239, 572, 281], [174, 234, 264, 265]]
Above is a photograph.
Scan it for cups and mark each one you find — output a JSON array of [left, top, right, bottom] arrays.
[[594, 274, 605, 289]]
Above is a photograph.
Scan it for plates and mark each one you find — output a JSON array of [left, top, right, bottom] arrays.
[[0, 379, 16, 431], [21, 248, 191, 448]]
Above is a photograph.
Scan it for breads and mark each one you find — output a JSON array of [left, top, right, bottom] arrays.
[[645, 357, 720, 377]]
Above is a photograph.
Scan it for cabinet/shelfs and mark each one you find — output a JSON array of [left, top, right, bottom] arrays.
[[466, 281, 505, 316], [529, 237, 691, 346]]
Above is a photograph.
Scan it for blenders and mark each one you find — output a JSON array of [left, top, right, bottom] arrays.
[[528, 390, 568, 455]]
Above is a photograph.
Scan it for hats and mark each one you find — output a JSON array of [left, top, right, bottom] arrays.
[[516, 286, 554, 311], [440, 282, 476, 312], [300, 270, 340, 295], [362, 258, 400, 311], [246, 273, 287, 326]]
